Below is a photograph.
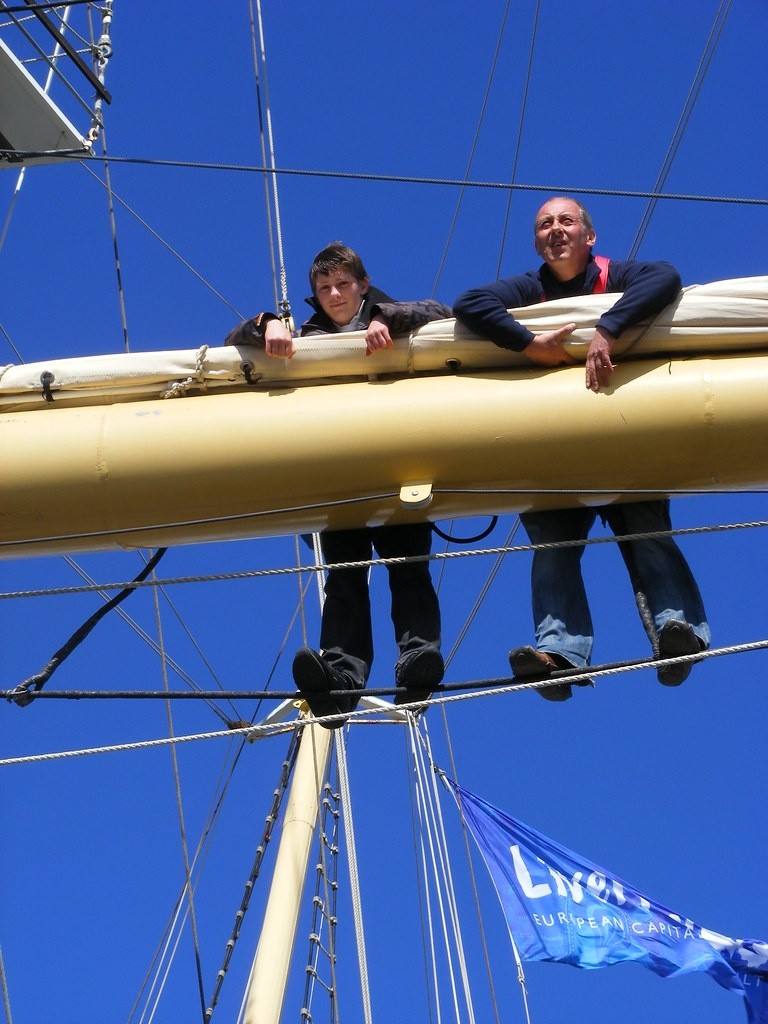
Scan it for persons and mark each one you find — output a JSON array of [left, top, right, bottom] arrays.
[[224, 240, 452, 731], [452, 196, 714, 702]]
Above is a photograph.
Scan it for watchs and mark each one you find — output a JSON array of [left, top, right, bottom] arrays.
[[251, 315, 277, 340]]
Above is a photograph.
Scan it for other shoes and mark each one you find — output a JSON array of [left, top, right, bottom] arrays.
[[656, 618, 699, 687], [508, 644, 572, 701], [293, 647, 355, 730], [393, 646, 446, 717]]
